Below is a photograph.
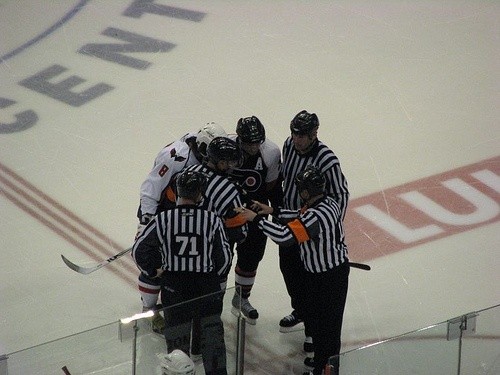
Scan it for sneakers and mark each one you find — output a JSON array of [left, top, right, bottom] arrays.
[[303, 357, 317, 370], [303, 337, 315, 351], [231, 295, 258, 325], [279, 310, 305, 333], [178, 349, 204, 363], [141, 308, 167, 338]]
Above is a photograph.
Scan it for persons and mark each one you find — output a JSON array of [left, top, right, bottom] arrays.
[[278, 110, 351, 328], [163, 136, 260, 360], [160, 349, 197, 375], [131, 170, 232, 375], [232, 115, 285, 320], [135, 121, 229, 335], [240, 165, 371, 375]]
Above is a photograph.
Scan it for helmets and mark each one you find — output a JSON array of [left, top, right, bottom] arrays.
[[290, 110, 319, 135], [196, 120, 228, 152], [236, 115, 265, 145], [160, 350, 196, 375], [293, 168, 326, 195], [208, 137, 242, 168], [176, 170, 210, 198]]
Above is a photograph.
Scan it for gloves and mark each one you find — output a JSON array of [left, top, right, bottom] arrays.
[[133, 225, 148, 245]]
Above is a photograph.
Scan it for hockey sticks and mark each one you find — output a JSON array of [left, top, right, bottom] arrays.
[[61, 366, 72, 375], [60, 245, 134, 275], [348, 261, 372, 272]]
